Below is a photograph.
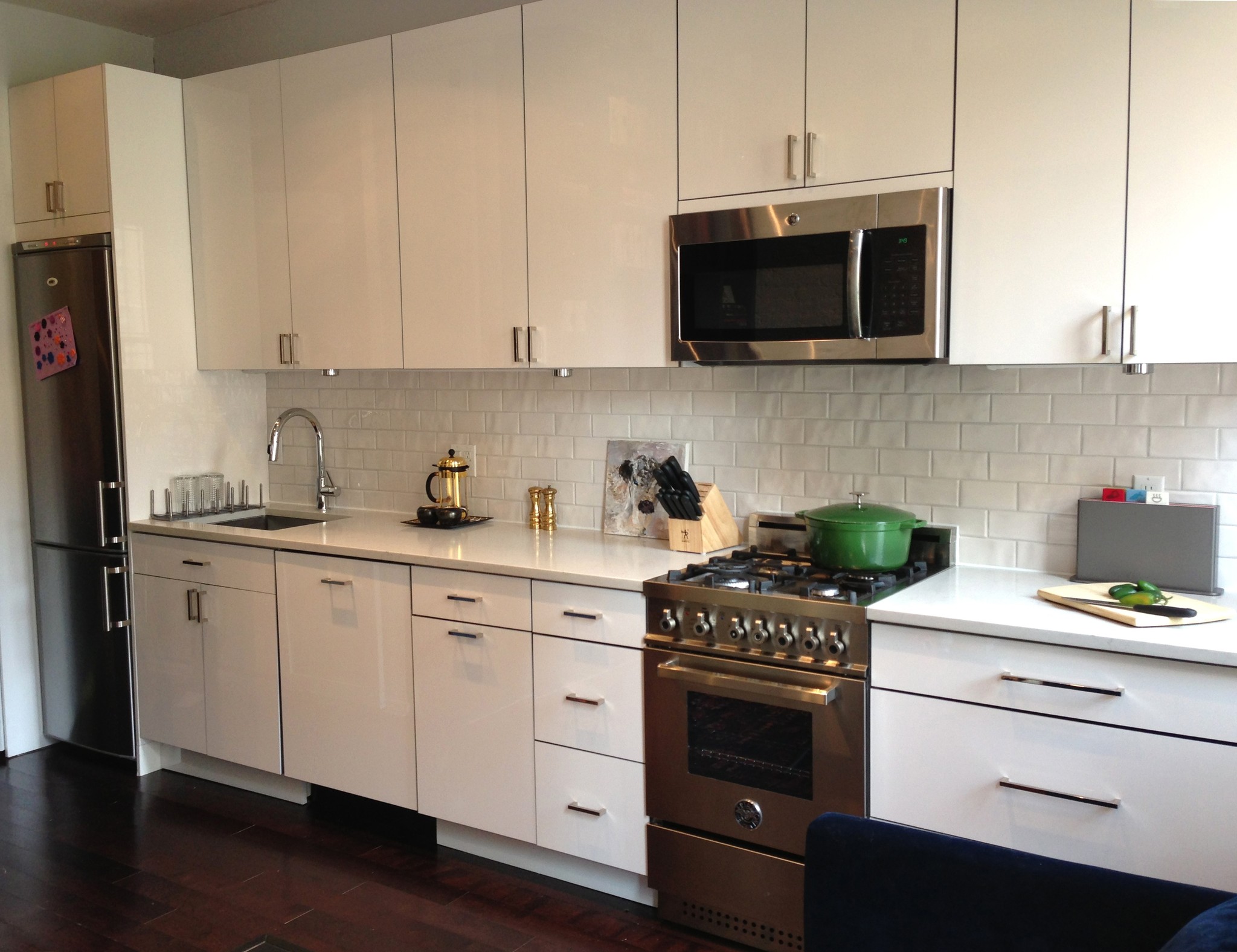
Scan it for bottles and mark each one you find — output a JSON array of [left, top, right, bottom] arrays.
[[528, 486, 544, 530], [542, 485, 557, 531]]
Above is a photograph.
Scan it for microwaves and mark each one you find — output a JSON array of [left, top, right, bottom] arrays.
[[662, 186, 956, 375]]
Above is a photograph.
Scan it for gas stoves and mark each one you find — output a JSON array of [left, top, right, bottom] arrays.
[[640, 511, 957, 681]]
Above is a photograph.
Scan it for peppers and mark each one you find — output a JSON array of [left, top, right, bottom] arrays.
[[1108, 579, 1173, 607]]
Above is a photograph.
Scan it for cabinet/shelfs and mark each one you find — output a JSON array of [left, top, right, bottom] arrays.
[[129, 531, 646, 878], [392, 0, 677, 372], [8, 64, 185, 224], [183, 33, 404, 370], [954, 2, 1230, 364], [869, 614, 1236, 896], [678, 2, 956, 198]]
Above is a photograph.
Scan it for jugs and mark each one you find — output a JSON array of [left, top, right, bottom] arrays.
[[425, 448, 469, 521]]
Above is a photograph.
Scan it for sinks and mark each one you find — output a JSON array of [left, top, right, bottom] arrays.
[[205, 514, 330, 533]]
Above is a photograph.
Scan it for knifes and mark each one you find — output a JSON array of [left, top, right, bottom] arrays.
[[1058, 594, 1198, 618], [651, 455, 703, 523]]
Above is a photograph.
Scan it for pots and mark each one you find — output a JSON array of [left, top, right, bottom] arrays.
[[794, 490, 928, 571]]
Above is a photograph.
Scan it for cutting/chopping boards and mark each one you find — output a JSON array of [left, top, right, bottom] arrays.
[[1038, 582, 1230, 629]]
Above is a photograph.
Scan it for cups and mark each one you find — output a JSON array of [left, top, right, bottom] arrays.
[[416, 504, 439, 524], [435, 505, 468, 526], [201, 471, 225, 512], [175, 474, 201, 514]]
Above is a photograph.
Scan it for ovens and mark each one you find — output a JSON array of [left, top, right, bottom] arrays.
[[636, 645, 872, 944]]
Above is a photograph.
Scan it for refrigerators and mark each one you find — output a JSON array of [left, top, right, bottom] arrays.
[[5, 226, 157, 757]]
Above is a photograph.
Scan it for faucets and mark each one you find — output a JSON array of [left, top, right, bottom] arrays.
[[266, 407, 342, 512]]
